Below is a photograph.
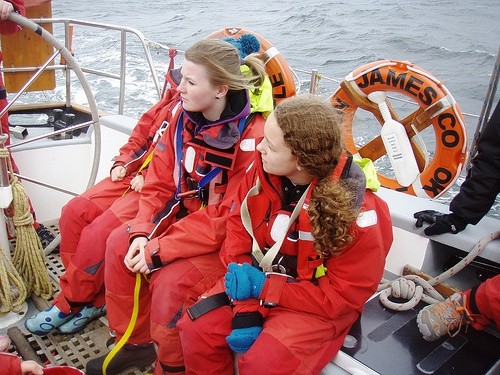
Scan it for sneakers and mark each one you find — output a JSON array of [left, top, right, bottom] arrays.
[[36, 224, 60, 253], [416, 293, 470, 341]]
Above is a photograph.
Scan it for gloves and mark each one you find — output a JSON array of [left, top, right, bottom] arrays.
[[226, 326, 262, 352], [414, 210, 459, 236], [225, 262, 265, 300]]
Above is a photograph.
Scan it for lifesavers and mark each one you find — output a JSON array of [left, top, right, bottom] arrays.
[[201, 27, 296, 110], [330, 59, 468, 199]]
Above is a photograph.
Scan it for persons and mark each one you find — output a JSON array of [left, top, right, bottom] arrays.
[[25, 68, 181, 336], [86, 39, 266, 375], [0, 0, 39, 238], [1, 352, 84, 375], [414, 103, 499, 341], [179, 94, 392, 375]]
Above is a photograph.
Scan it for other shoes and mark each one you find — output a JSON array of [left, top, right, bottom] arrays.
[[58, 304, 106, 334], [24, 304, 75, 335], [86, 342, 157, 375]]
